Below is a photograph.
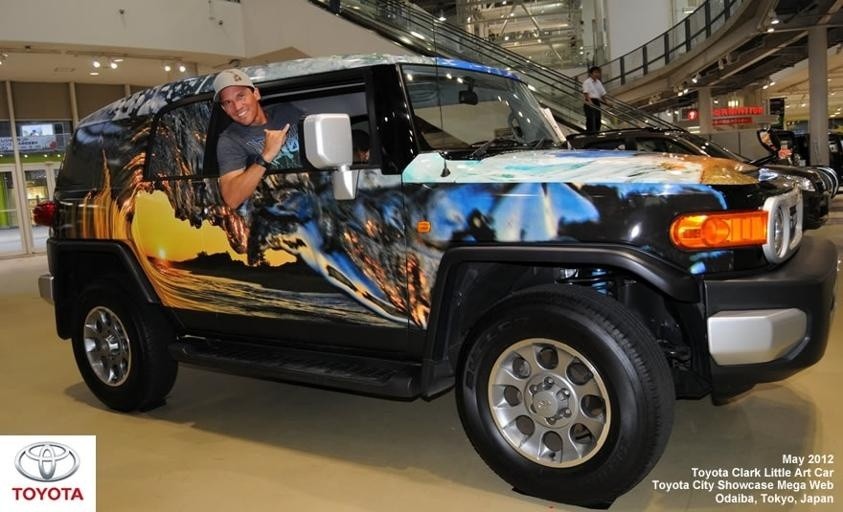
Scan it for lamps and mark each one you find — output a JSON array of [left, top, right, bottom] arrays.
[[690, 73, 702, 83], [90, 54, 122, 74], [779, 94, 810, 112], [671, 82, 688, 95], [437, 6, 450, 23], [161, 56, 189, 73], [758, 76, 775, 89], [756, 10, 780, 37]]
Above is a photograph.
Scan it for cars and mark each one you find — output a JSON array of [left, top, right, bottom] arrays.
[[549, 125, 833, 235]]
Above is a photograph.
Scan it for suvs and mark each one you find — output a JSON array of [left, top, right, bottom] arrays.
[[33, 11, 842, 505]]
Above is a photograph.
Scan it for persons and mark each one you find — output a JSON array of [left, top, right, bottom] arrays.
[[210, 68, 308, 213], [581, 66, 613, 136]]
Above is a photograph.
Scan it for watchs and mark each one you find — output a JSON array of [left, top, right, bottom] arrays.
[[254, 155, 272, 169], [351, 129, 370, 162]]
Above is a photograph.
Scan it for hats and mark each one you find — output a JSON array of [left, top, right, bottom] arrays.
[[213, 68, 255, 102]]
